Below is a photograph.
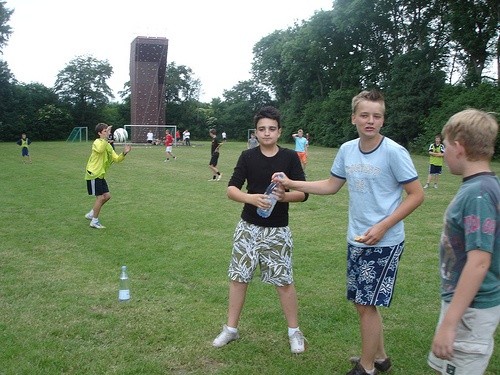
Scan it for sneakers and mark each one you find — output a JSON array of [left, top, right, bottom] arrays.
[[85, 213, 92, 220], [213, 325, 238, 347], [289, 330, 308, 354], [351, 356, 392, 372], [347, 362, 374, 375], [90, 219, 106, 229]]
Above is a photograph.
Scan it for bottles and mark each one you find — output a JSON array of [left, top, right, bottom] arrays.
[[257, 174, 284, 218], [118, 266, 130, 301]]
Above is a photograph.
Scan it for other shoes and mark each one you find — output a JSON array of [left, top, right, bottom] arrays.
[[207, 177, 216, 181], [165, 159, 169, 162], [217, 173, 222, 182], [424, 183, 429, 188], [174, 157, 177, 161], [434, 184, 437, 188]]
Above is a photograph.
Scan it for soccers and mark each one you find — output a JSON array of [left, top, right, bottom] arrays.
[[113, 127, 128, 143]]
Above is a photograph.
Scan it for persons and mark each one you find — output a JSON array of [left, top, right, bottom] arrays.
[[269, 88, 425, 375], [163, 129, 177, 163], [213, 106, 309, 354], [426, 107, 500, 375], [208, 128, 224, 182], [145, 129, 154, 149], [83, 122, 132, 229], [221, 130, 227, 143], [107, 124, 115, 150], [175, 129, 182, 146], [246, 132, 259, 150], [182, 129, 190, 146], [17, 133, 32, 164], [423, 133, 445, 189], [291, 129, 309, 177]]
